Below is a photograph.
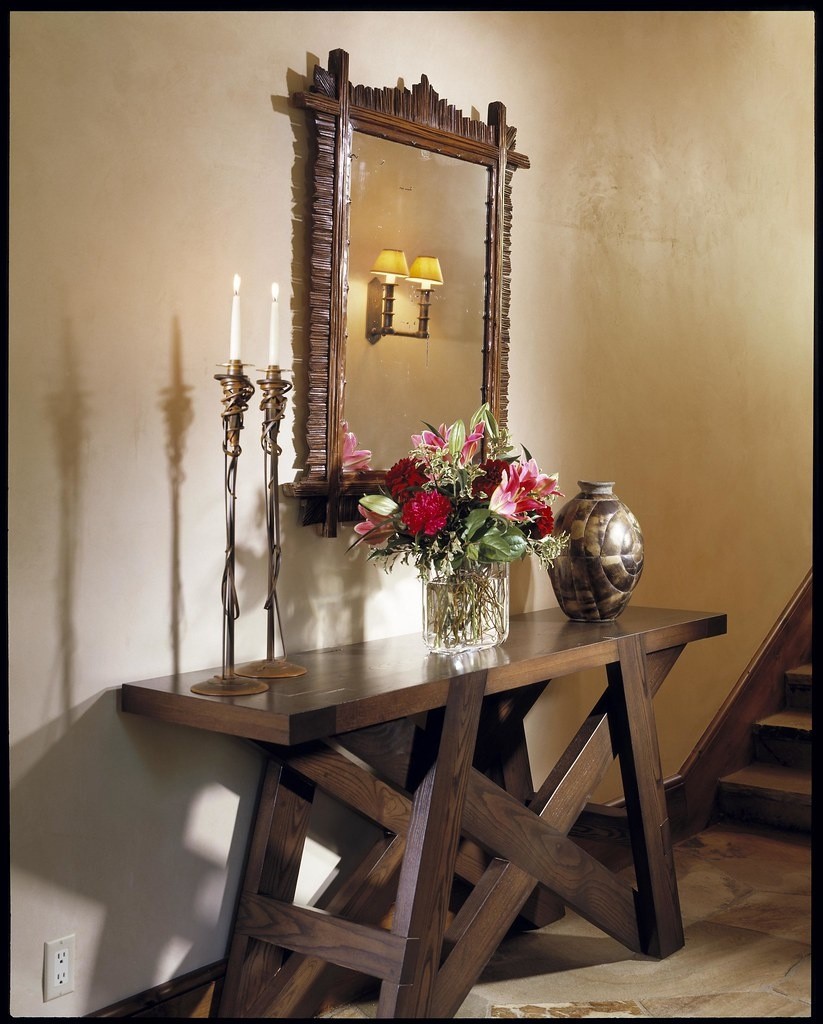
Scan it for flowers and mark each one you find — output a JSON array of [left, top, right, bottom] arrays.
[[344, 403, 571, 648], [343, 420, 372, 473]]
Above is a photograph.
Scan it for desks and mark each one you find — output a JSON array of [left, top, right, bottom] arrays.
[[122, 607, 727, 1020]]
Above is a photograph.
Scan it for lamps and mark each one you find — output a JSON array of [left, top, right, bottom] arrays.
[[365, 249, 444, 345]]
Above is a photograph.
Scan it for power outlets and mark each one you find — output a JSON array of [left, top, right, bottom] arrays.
[[44, 934, 75, 1003]]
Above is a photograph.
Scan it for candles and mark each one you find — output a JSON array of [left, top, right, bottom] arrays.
[[269, 282, 281, 365], [230, 273, 243, 360]]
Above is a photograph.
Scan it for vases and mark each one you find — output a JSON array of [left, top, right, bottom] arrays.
[[422, 552, 512, 654], [548, 481, 644, 622]]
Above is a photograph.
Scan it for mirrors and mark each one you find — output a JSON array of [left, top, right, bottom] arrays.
[[282, 48, 530, 539]]
